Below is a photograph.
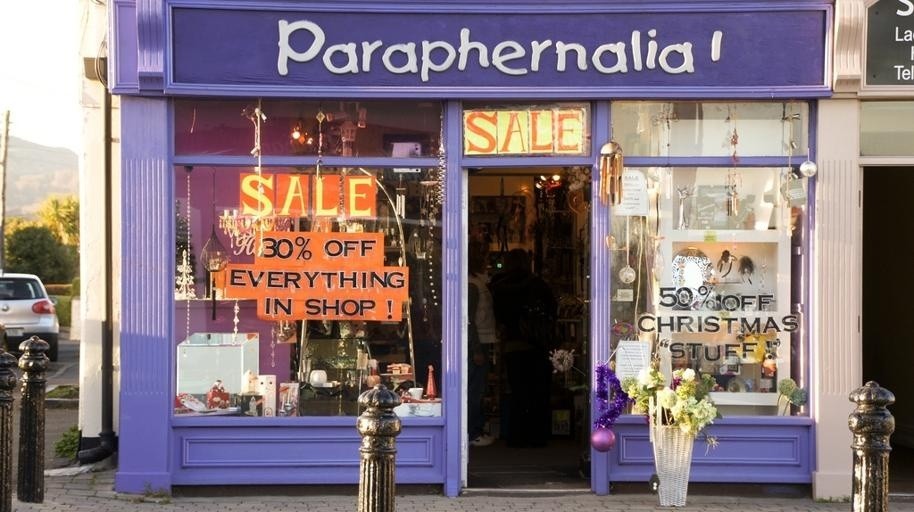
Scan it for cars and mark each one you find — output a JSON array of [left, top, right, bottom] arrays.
[[704, 375, 754, 392], [0, 273, 59, 361], [368, 340, 407, 373]]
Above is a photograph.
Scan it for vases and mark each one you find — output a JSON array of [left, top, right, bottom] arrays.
[[651, 425, 700, 509]]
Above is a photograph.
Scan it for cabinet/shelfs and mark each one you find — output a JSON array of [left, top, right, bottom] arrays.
[[647, 119, 792, 419], [174, 324, 272, 417]]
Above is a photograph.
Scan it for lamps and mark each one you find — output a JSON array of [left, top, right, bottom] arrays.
[[530, 171, 564, 192]]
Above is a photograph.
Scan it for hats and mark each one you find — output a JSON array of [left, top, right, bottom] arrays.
[[469, 223, 494, 245]]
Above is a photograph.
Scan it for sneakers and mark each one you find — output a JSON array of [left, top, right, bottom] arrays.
[[469, 432, 496, 446]]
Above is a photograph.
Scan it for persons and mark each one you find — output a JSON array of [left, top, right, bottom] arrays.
[[466, 237, 498, 449], [482, 246, 560, 451]]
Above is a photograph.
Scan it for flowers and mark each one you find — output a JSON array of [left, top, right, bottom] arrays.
[[631, 360, 720, 458]]
